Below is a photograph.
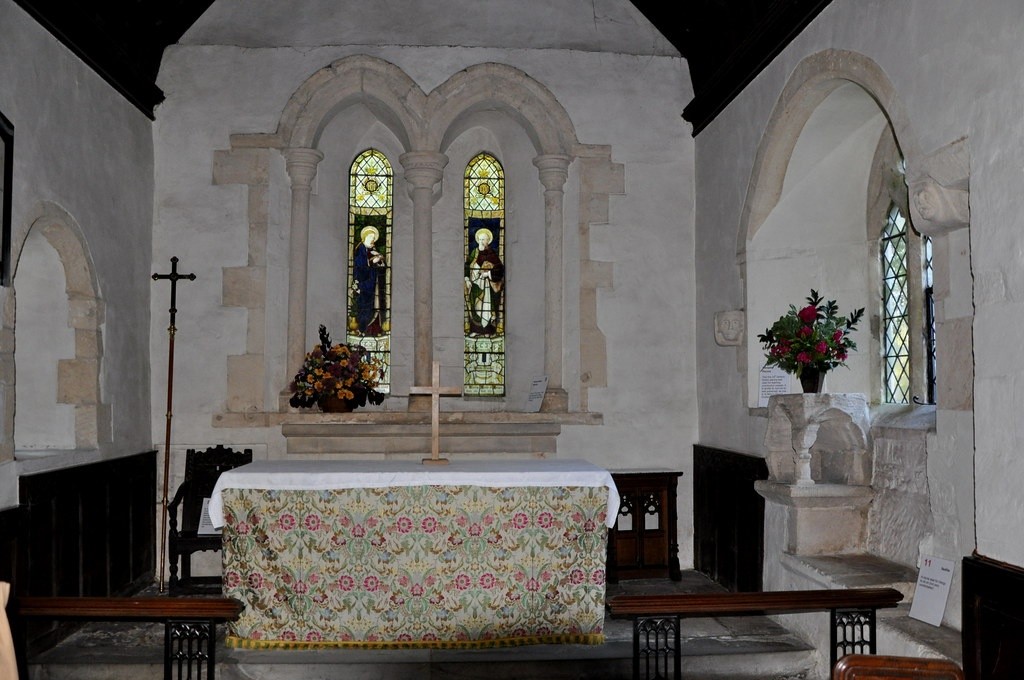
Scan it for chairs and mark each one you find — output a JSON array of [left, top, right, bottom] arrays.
[[164, 444, 252, 598]]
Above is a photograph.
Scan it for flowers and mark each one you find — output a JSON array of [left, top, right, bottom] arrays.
[[288, 324, 386, 409], [758, 287, 866, 380]]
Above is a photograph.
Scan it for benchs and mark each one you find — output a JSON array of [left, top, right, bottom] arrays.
[[11, 597, 245, 680], [605, 588, 903, 680], [834, 556, 1023, 680]]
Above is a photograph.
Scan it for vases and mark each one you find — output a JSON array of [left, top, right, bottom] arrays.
[[315, 395, 358, 414], [793, 367, 828, 394]]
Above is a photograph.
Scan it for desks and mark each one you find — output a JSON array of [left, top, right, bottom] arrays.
[[210, 459, 621, 649]]
